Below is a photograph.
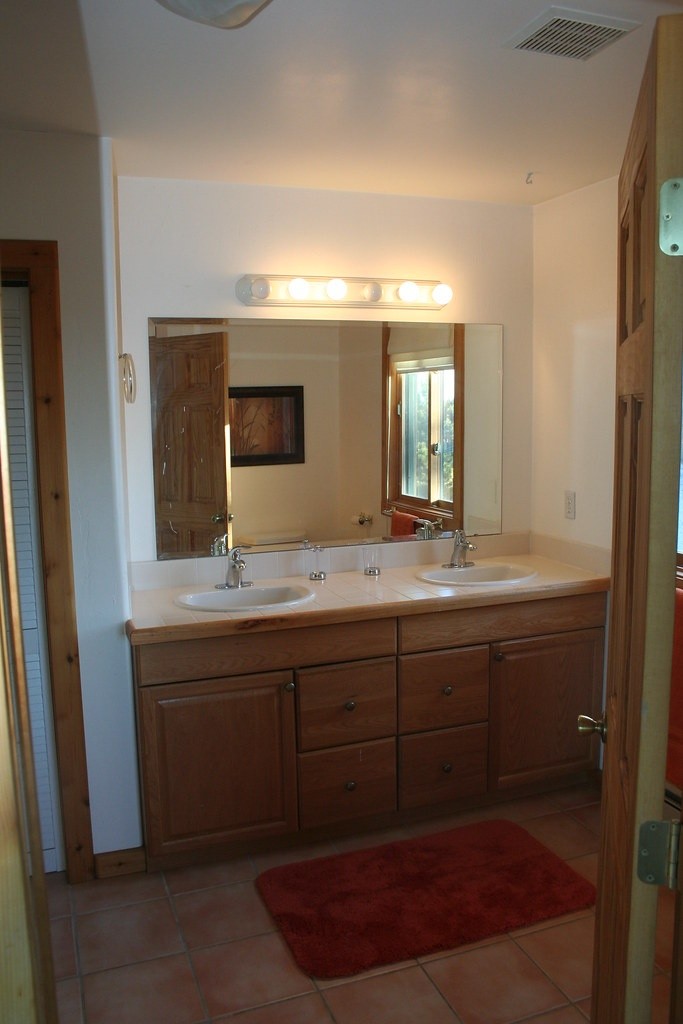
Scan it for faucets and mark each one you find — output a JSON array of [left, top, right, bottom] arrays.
[[450, 528, 480, 566], [209, 531, 229, 557], [225, 543, 255, 589], [416, 518, 439, 540]]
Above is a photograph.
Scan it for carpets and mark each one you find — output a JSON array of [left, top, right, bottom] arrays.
[[254, 820, 598, 979]]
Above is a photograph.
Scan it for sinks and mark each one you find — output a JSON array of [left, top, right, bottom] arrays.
[[415, 560, 541, 586], [175, 575, 316, 617]]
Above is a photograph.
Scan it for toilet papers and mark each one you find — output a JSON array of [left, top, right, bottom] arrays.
[[350, 515, 365, 527]]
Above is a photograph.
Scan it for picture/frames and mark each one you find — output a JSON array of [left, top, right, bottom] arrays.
[[228, 386, 305, 467]]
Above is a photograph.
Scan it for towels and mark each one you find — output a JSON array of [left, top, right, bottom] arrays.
[[390, 510, 421, 537]]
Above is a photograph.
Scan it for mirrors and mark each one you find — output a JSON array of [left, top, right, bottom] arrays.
[[148, 317, 504, 562]]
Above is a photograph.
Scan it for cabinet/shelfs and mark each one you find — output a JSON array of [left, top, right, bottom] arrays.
[[133, 616, 398, 873], [397, 590, 601, 824]]
